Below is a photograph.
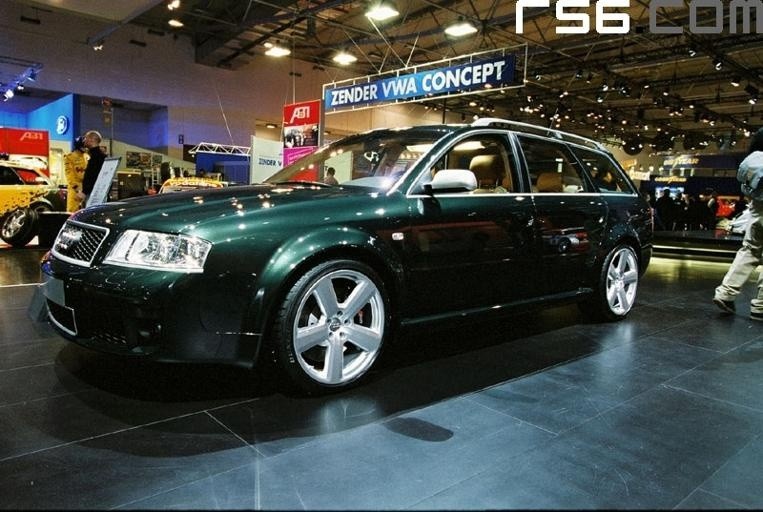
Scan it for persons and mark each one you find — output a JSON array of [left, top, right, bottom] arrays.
[[324, 168, 338, 185], [0, 152, 9, 161], [644, 189, 719, 231], [63, 136, 88, 212], [77, 131, 108, 208], [714, 129, 763, 319]]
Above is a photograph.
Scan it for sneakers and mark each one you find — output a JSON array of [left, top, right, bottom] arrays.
[[712, 297, 736, 314], [750, 312, 763, 321]]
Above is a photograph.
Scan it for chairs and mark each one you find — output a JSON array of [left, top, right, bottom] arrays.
[[534, 172, 565, 193], [465, 155, 509, 194]]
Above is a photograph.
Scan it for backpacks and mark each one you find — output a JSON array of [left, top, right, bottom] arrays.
[[737, 150, 763, 200]]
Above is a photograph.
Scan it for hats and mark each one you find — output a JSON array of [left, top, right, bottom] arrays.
[[704, 187, 714, 196]]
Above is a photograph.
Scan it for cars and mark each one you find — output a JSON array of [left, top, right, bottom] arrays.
[[38, 116, 657, 397], [0, 160, 68, 218], [148, 176, 223, 196]]
